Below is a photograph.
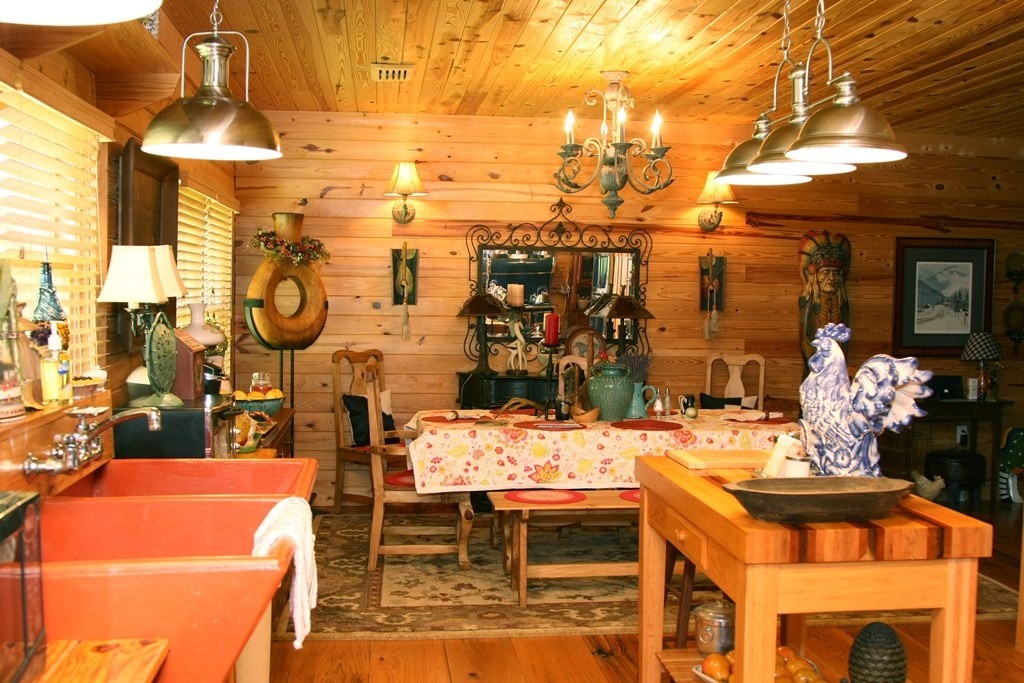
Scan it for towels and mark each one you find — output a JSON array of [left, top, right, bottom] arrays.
[[253, 495, 320, 650]]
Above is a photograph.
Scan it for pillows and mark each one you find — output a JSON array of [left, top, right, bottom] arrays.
[[343, 388, 400, 448]]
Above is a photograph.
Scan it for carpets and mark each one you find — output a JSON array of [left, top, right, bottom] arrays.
[[270, 511, 1019, 642]]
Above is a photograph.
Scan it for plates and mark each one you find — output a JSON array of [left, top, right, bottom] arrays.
[[240, 422, 277, 453]]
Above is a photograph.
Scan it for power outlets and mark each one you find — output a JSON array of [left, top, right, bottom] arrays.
[[957, 426, 969, 443]]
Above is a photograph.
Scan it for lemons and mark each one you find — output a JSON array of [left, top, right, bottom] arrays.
[[220, 389, 284, 400]]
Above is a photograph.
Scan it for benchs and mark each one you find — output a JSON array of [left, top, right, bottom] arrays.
[[485, 490, 684, 608]]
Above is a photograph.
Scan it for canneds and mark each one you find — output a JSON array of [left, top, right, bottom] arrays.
[[555, 395, 570, 420]]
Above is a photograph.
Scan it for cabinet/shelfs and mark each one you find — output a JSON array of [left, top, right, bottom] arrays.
[[456, 371, 560, 512]]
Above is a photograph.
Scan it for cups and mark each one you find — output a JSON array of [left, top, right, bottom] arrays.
[[678, 394, 695, 414]]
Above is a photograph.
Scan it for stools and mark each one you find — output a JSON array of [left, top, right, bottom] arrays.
[[925, 450, 986, 511]]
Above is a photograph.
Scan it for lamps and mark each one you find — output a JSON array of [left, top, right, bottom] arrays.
[[456, 293, 509, 375], [961, 332, 1000, 402], [582, 294, 619, 340], [97, 244, 187, 408], [0, 0, 905, 229], [605, 295, 656, 357]]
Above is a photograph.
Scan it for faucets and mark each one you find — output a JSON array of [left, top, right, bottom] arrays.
[[23, 400, 163, 478]]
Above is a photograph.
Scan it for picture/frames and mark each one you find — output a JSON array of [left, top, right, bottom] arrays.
[[565, 328, 607, 358], [892, 237, 996, 358]]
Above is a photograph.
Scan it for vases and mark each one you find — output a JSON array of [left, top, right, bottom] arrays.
[[186, 304, 223, 345]]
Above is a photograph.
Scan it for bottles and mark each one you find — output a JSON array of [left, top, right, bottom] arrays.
[[587, 364, 634, 422], [249, 373, 273, 394], [182, 303, 224, 345], [653, 387, 671, 418], [27, 262, 69, 349]]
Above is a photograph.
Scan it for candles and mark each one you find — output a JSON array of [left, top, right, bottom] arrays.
[[511, 285, 523, 307], [545, 314, 560, 344]]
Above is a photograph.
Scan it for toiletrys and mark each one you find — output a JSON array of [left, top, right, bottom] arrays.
[[38, 319, 78, 405]]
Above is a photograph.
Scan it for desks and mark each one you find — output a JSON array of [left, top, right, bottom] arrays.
[[878, 398, 1014, 513], [401, 408, 802, 576], [634, 457, 994, 683]]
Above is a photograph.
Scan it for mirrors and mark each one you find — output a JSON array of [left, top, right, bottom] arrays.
[[456, 199, 662, 361]]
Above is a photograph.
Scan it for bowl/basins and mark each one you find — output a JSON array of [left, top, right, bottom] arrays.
[[693, 599, 735, 659], [235, 397, 285, 421]]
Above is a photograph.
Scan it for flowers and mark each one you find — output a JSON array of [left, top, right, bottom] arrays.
[[246, 227, 329, 264]]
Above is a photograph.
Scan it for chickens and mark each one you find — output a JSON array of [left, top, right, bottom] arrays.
[[796, 321, 934, 479]]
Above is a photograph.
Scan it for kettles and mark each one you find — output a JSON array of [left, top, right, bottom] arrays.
[[532, 286, 549, 305]]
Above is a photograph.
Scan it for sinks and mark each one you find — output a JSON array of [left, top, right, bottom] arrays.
[[46, 454, 320, 506], [12, 491, 295, 682]]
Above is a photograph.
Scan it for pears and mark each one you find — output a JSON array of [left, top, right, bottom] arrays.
[[703, 646, 828, 683]]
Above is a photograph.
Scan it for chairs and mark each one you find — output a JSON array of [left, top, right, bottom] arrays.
[[705, 353, 766, 411], [332, 350, 474, 572]]
